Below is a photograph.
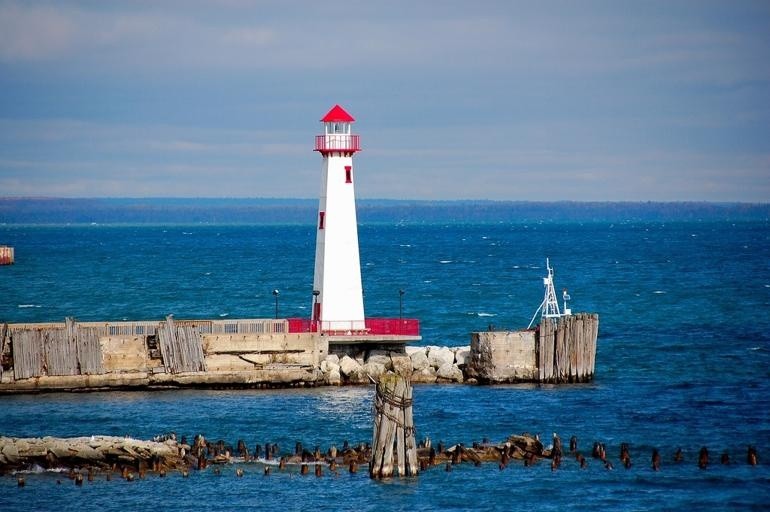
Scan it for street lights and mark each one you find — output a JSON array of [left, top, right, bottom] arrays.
[[399, 290, 404, 318], [312, 291, 319, 320], [273, 289, 279, 319]]
